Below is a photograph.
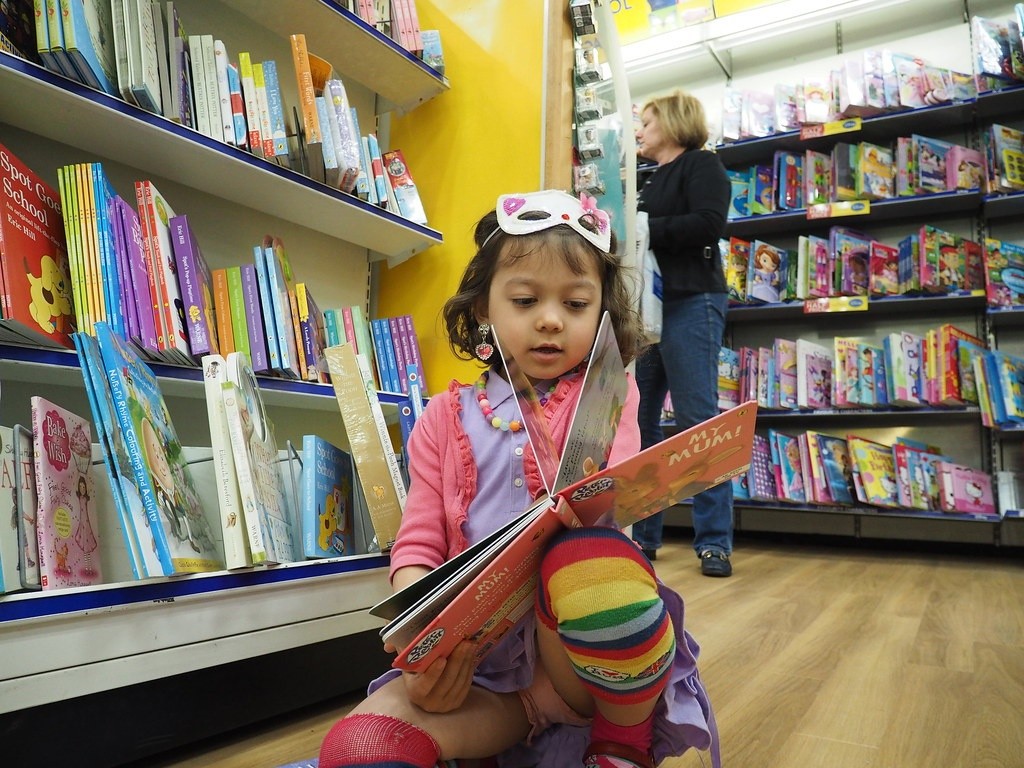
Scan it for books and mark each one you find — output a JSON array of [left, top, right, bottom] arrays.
[[330, 0, 446, 76], [726, 123, 1024, 220], [718, 225, 1024, 307], [660, 322, 1024, 432], [632, 2, 1024, 169], [0, 141, 430, 398], [0, 320, 424, 597], [607, 0, 790, 47], [368, 310, 757, 674], [732, 427, 997, 513], [0, 0, 428, 226]]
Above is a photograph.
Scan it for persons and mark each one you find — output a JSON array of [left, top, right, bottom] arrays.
[[317, 189, 721, 768], [631, 90, 733, 578]]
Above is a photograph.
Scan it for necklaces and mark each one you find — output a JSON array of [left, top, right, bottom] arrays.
[[478, 361, 583, 432]]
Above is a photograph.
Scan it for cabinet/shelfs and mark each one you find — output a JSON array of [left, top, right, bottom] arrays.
[[596, 0, 1024, 548], [0, 0, 446, 768]]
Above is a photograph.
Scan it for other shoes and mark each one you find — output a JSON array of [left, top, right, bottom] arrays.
[[630, 537, 657, 561], [583, 740, 657, 768], [701, 542, 733, 577]]
